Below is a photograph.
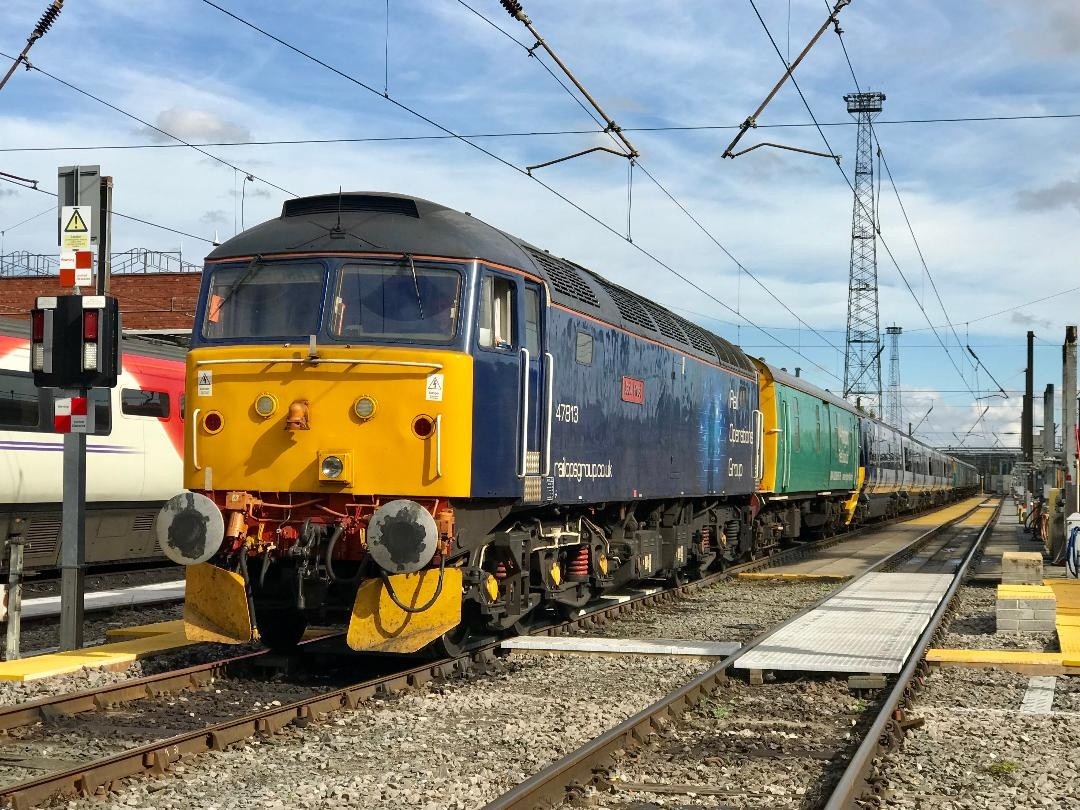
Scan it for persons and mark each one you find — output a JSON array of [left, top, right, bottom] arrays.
[[432, 292, 503, 346]]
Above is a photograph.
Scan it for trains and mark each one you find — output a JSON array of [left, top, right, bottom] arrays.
[[156, 190, 980, 664], [0, 334, 187, 584]]
